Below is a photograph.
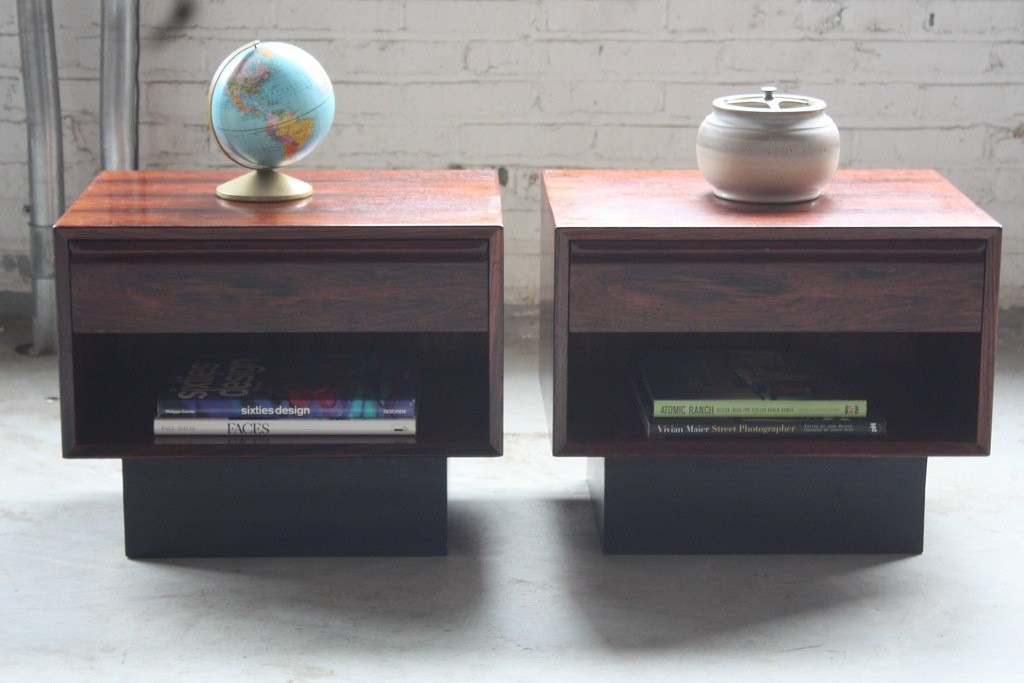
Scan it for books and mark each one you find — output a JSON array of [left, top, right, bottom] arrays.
[[156, 351, 423, 418], [639, 347, 869, 419], [627, 373, 888, 442], [153, 418, 420, 435]]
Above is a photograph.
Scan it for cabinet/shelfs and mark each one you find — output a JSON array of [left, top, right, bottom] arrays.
[[48, 169, 507, 461], [537, 170, 1003, 456]]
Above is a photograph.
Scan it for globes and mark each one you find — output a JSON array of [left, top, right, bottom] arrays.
[[204, 40, 336, 204]]
